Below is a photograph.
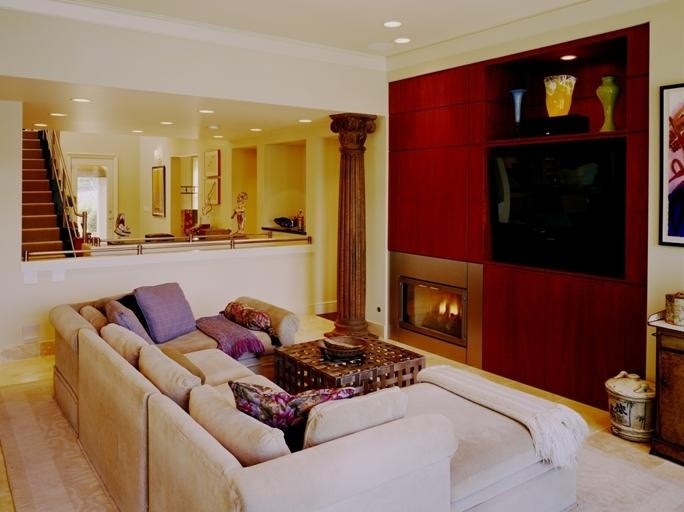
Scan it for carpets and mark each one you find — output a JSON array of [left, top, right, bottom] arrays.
[[1, 375, 683, 512]]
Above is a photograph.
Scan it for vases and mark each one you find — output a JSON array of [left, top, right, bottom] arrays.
[[595, 73, 621, 133]]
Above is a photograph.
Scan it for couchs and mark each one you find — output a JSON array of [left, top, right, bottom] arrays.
[[50, 282, 592, 512]]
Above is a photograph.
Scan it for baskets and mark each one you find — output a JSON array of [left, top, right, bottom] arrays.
[[318, 336, 367, 357]]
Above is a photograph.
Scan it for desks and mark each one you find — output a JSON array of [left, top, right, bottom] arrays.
[[260, 225, 306, 241]]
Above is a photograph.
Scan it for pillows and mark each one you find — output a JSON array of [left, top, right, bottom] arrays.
[[133, 283, 198, 342], [232, 381, 291, 435], [163, 348, 208, 386], [104, 300, 156, 345], [224, 302, 270, 333], [283, 386, 364, 432]]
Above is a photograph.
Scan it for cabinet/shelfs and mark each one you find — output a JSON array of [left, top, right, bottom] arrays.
[[645, 307, 684, 467], [483, 21, 650, 411]]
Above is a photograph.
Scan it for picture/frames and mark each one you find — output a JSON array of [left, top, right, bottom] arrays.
[[659, 81, 684, 249], [150, 165, 167, 219]]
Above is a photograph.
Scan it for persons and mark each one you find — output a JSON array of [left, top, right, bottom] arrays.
[[229, 191, 248, 236]]
